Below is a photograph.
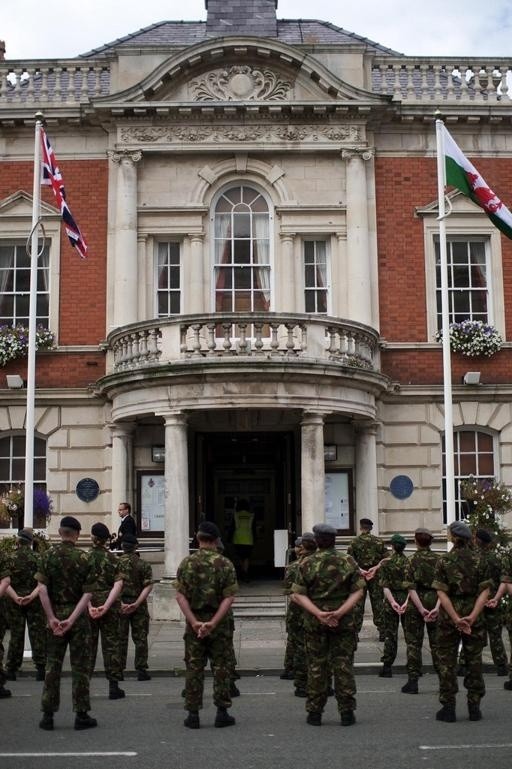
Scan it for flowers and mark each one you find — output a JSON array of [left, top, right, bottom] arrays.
[[0, 485, 54, 523], [0, 324, 55, 368], [436, 319, 505, 358], [460, 476, 512, 529]]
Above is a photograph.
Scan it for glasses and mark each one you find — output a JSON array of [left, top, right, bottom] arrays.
[[119, 508, 127, 511]]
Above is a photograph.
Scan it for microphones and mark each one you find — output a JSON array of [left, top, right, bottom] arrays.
[[110, 533, 116, 542]]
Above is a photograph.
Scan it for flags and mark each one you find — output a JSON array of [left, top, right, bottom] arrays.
[[441, 124, 512, 240], [38, 126, 89, 261]]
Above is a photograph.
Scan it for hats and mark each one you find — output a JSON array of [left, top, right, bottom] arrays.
[[415, 527, 434, 538], [312, 523, 337, 536], [302, 532, 317, 543], [391, 534, 407, 545], [91, 522, 110, 537], [295, 537, 303, 547], [475, 529, 491, 542], [122, 534, 139, 544], [198, 522, 220, 537], [359, 518, 373, 525], [60, 516, 81, 531], [18, 530, 34, 541], [450, 521, 473, 539]]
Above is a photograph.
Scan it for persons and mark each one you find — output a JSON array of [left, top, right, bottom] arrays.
[[431, 521, 490, 721], [291, 523, 366, 725], [171, 500, 257, 728], [379, 534, 410, 678], [282, 532, 335, 698], [0, 502, 154, 730], [457, 529, 510, 677], [346, 519, 387, 642], [401, 527, 458, 694], [504, 554, 510, 691]]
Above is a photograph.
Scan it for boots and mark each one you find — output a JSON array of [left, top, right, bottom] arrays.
[[184, 711, 200, 729], [215, 707, 236, 728], [39, 712, 55, 731], [75, 713, 98, 730], [434, 704, 456, 722], [1, 686, 12, 699], [504, 680, 512, 691], [280, 671, 334, 696], [306, 712, 322, 726], [400, 681, 418, 694], [36, 665, 46, 681], [109, 681, 125, 700], [379, 664, 392, 678], [341, 711, 355, 726], [468, 705, 482, 721], [137, 670, 152, 680]]
